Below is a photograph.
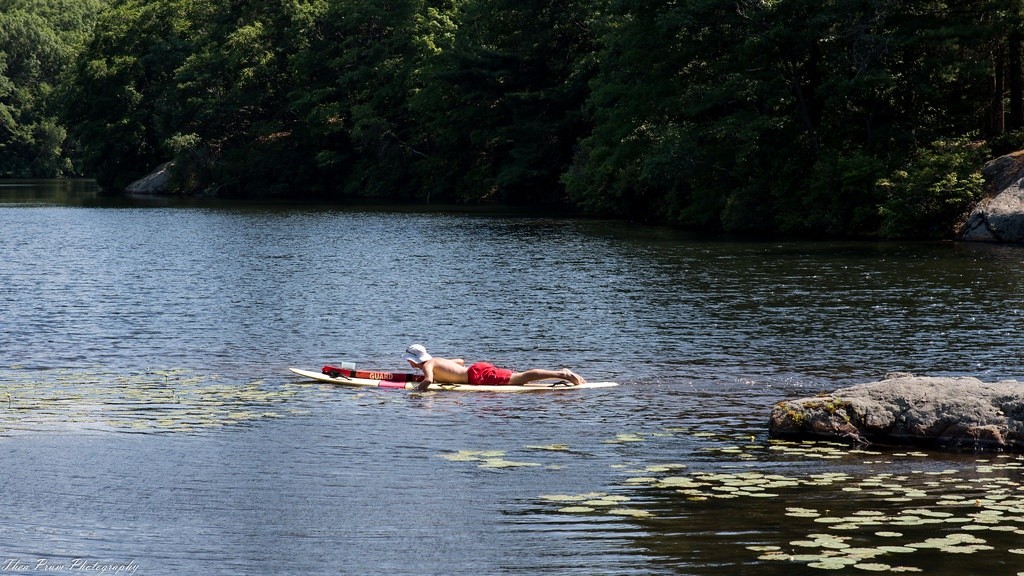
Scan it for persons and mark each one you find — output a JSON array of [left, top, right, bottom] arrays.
[[401, 344, 587, 390]]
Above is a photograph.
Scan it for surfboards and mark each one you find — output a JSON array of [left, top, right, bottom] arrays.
[[289, 366, 619, 392]]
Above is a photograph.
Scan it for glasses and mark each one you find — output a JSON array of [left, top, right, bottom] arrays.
[[406, 349, 417, 356]]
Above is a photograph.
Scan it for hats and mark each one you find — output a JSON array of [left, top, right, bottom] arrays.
[[402, 344, 432, 364]]
